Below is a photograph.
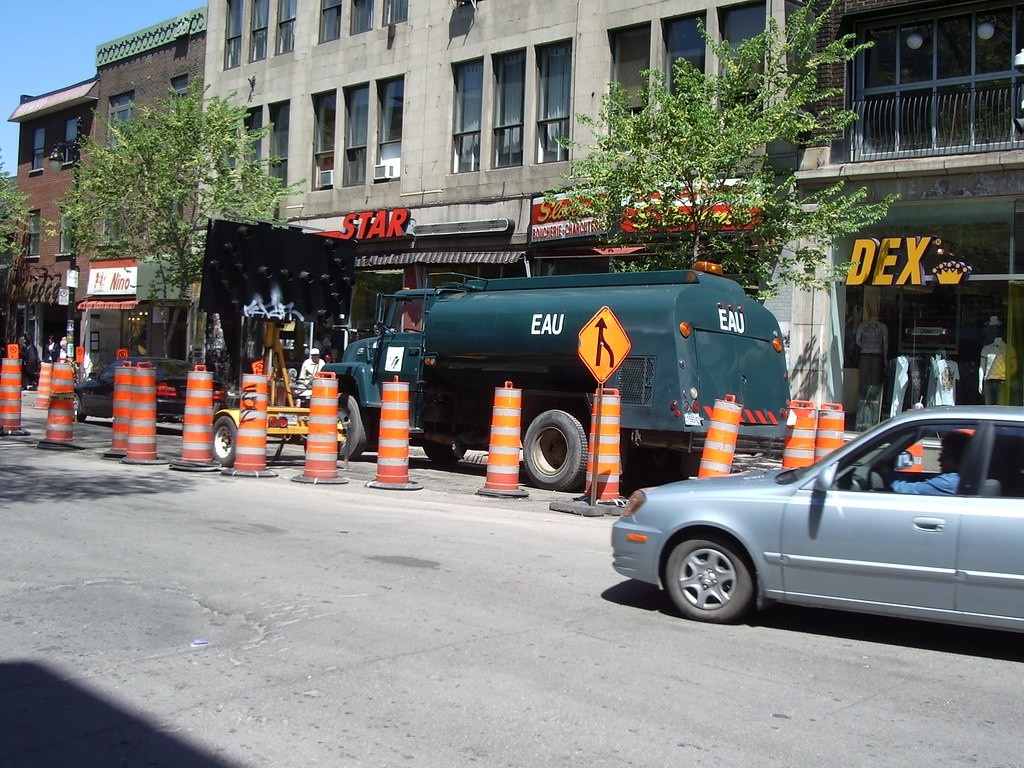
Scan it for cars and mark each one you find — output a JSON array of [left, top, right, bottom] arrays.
[[71, 356, 226, 422], [609, 405, 1024, 637]]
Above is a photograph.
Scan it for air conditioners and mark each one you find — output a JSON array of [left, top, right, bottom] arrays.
[[374, 164, 395, 180], [318, 170, 333, 187]]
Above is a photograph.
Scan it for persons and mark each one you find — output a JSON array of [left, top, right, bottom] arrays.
[[982, 316, 1005, 344], [44, 336, 58, 363], [56, 337, 67, 362], [299, 349, 325, 380], [928, 351, 960, 406], [881, 431, 971, 496], [856, 317, 888, 383], [978, 338, 1017, 405]]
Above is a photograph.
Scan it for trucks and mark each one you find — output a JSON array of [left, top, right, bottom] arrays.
[[318, 260, 792, 495]]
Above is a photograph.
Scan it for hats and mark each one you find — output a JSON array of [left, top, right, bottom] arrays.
[[309, 348, 320, 355]]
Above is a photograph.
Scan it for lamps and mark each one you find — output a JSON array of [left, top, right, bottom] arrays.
[[977, 14, 997, 40], [907, 28, 922, 50]]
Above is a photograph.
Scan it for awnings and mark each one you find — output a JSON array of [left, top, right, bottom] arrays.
[[355, 250, 532, 268], [8, 80, 100, 123]]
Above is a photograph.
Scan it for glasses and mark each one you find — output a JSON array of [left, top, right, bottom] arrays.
[[939, 451, 955, 459]]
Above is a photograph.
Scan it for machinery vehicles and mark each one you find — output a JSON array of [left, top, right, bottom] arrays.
[[198, 217, 357, 468]]
